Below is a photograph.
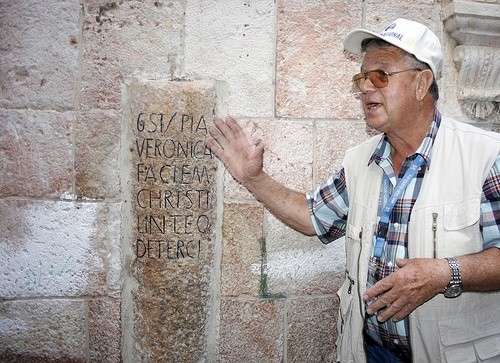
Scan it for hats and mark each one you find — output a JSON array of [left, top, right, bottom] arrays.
[[343, 19, 443, 81]]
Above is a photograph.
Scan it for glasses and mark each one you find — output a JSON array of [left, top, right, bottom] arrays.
[[353, 69, 422, 92]]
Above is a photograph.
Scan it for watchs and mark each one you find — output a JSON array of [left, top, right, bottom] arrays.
[[440, 256, 463, 299]]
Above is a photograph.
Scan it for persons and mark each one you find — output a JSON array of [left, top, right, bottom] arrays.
[[203, 17, 500, 363]]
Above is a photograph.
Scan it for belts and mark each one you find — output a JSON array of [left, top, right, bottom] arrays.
[[363, 329, 412, 363]]
